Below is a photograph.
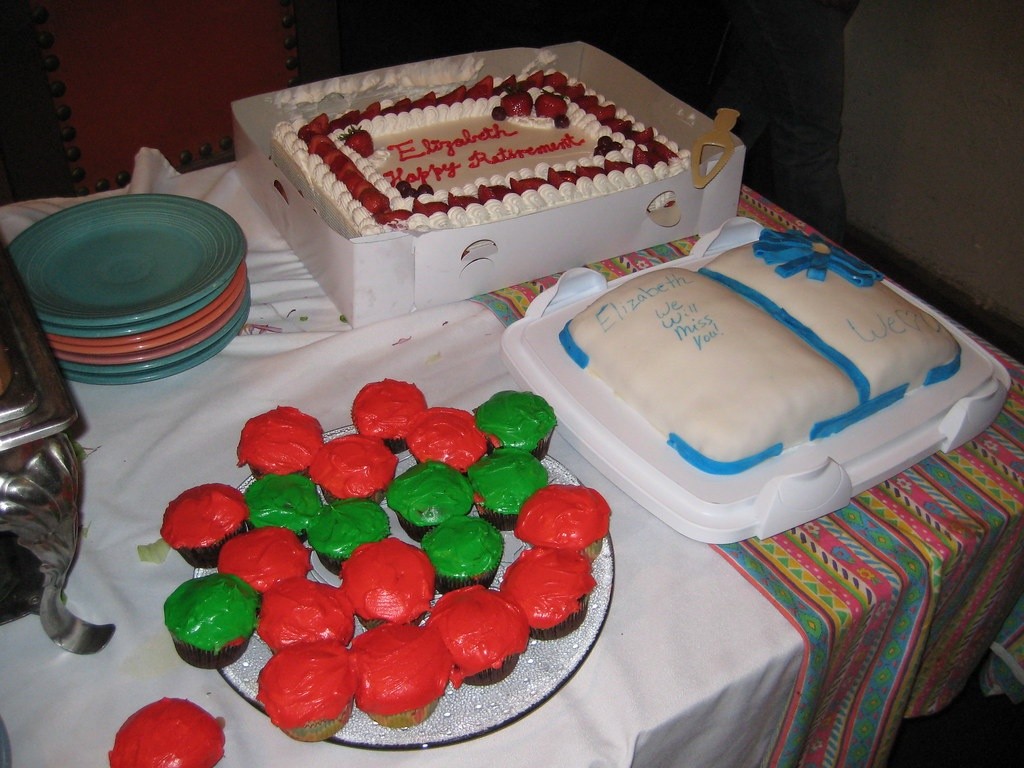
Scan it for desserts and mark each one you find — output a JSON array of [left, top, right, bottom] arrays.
[[108, 697, 225, 768], [158, 377, 612, 743]]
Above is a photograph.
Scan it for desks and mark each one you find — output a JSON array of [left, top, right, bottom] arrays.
[[0, 173, 1023, 768]]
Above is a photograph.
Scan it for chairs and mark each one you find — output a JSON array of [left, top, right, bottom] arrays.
[[0, 0, 342, 204]]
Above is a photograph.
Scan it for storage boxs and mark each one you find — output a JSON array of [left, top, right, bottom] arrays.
[[230, 41, 746, 329]]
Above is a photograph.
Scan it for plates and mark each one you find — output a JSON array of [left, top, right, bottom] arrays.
[[4, 193, 250, 386], [193, 422, 615, 753]]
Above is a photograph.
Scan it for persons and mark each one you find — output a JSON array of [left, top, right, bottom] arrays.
[[702, 0, 861, 251]]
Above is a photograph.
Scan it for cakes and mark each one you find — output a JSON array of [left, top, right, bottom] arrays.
[[268, 69, 694, 240], [559, 228, 961, 476]]
